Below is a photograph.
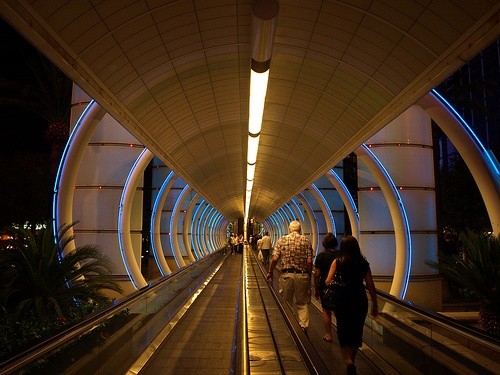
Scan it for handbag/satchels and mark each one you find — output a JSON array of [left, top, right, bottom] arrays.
[[258, 250, 264, 261]]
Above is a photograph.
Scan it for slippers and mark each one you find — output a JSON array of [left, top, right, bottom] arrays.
[[323, 335, 333, 342]]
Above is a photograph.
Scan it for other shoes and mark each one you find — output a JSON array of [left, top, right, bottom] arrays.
[[302, 327, 308, 336], [347, 364, 356, 375]]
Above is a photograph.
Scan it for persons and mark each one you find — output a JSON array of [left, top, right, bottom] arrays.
[[228, 231, 272, 265], [266, 221, 315, 334], [313, 232, 338, 343], [325, 235, 379, 375]]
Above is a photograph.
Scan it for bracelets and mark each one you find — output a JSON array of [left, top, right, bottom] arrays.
[[372, 299, 377, 302], [269, 272, 272, 275]]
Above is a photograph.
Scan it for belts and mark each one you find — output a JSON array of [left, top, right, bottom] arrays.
[[282, 269, 308, 273]]
